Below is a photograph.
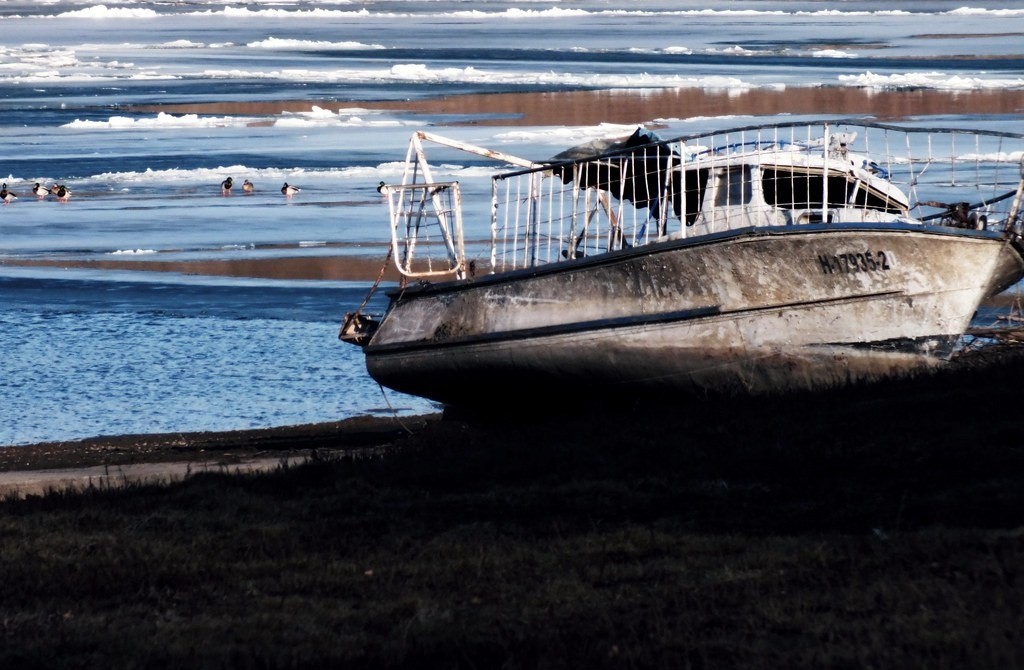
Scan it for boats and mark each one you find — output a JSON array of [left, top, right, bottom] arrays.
[[339, 119, 1023, 430]]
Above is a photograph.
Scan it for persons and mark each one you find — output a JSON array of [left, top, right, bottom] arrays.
[[377, 181, 397, 194], [0, 183, 15, 201], [281, 182, 299, 194], [221, 177, 233, 193], [33, 183, 70, 198], [242, 180, 254, 192]]
